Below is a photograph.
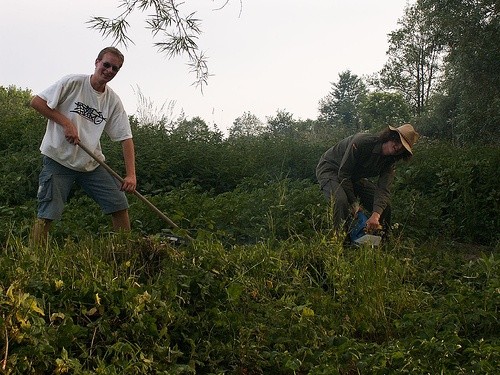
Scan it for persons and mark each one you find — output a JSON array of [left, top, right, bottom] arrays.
[[31, 46, 137, 247], [316, 124, 419, 252]]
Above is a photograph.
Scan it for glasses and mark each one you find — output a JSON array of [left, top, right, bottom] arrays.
[[97, 59, 119, 72]]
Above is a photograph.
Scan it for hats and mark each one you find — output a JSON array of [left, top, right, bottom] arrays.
[[387, 121, 420, 157]]
